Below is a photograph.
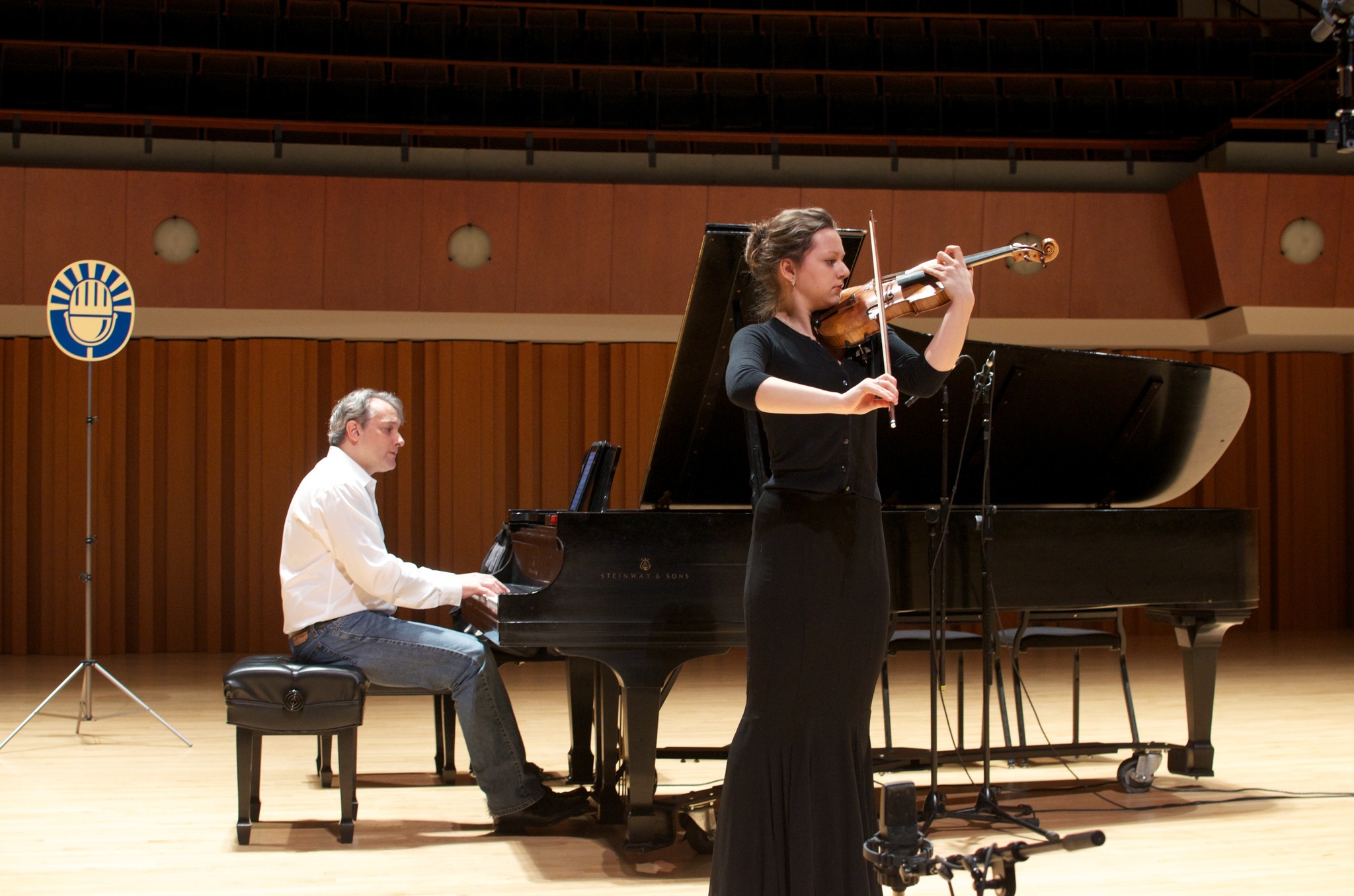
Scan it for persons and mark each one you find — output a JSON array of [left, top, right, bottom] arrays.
[[279, 387, 588, 835], [709, 207, 975, 895]]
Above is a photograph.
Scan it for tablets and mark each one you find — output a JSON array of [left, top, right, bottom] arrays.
[[567, 442, 602, 513]]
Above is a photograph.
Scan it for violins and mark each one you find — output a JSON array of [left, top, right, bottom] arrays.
[[811, 237, 1059, 361]]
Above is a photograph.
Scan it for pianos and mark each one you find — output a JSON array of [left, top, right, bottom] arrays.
[[451, 223, 1261, 853]]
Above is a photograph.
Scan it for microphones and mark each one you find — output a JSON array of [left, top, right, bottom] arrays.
[[877, 781, 919, 896], [904, 355, 965, 407], [972, 352, 995, 405]]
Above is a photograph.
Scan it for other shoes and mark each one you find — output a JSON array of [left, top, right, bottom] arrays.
[[492, 786, 589, 835]]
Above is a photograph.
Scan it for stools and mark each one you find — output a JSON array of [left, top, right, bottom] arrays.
[[299, 653, 472, 797], [217, 647, 368, 847]]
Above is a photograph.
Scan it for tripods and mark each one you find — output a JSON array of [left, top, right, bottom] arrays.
[[918, 367, 1059, 844]]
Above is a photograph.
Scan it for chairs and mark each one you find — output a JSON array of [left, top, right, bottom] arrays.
[[991, 598, 1146, 758], [863, 602, 991, 771]]
